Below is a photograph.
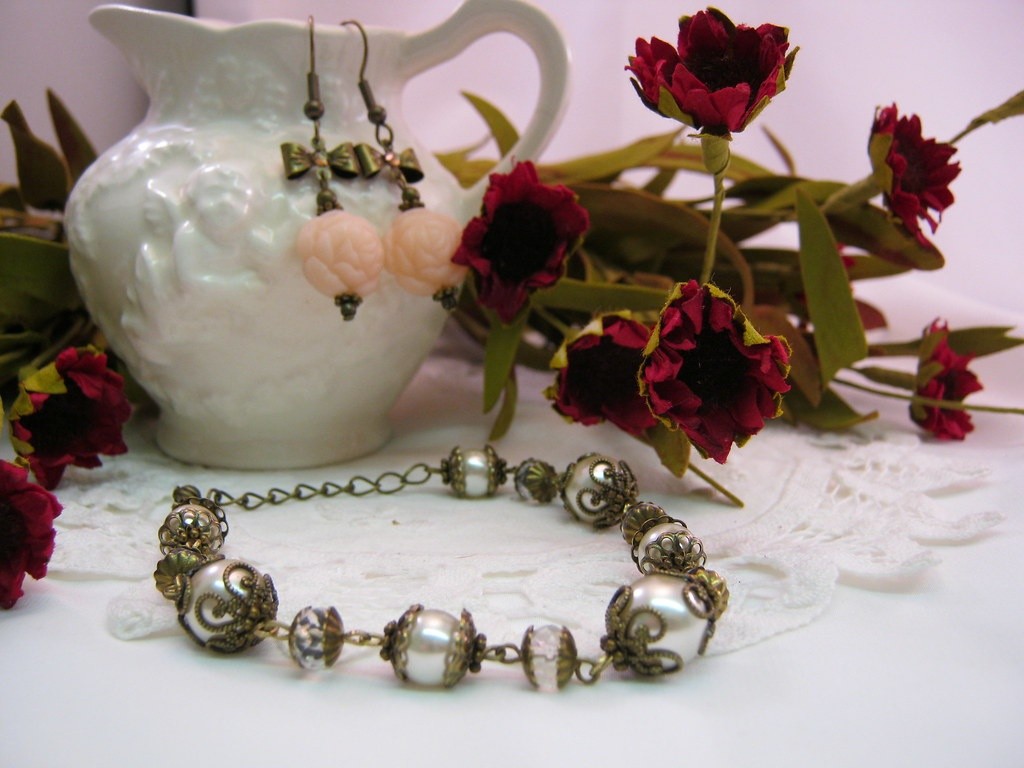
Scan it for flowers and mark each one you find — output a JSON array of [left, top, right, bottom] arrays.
[[0, 89, 146, 611], [436, 6, 1024, 511]]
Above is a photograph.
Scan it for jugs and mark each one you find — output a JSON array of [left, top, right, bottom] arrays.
[[63, 0, 576, 470]]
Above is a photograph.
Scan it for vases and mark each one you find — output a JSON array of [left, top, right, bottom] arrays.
[[61, 0, 576, 470]]
